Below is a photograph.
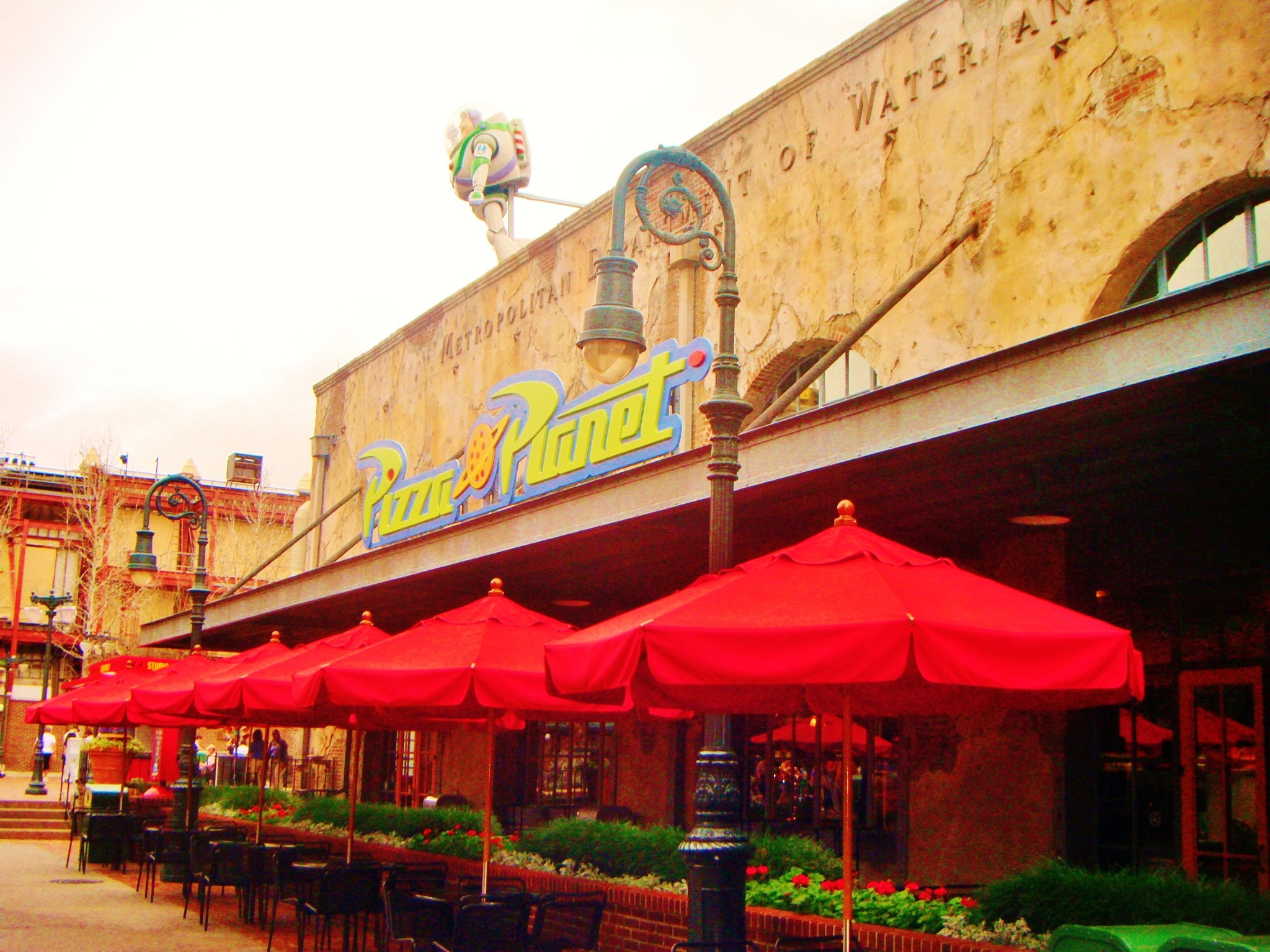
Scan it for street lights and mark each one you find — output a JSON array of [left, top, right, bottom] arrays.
[[23, 591, 79, 794], [574, 145, 752, 951], [126, 473, 211, 884]]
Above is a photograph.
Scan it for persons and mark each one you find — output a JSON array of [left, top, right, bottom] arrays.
[[84, 728, 94, 739], [32, 724, 56, 782], [62, 726, 80, 747], [755, 740, 856, 818], [195, 728, 288, 790]]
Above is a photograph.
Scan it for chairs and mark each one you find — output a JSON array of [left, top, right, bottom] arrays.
[[58, 796, 871, 952]]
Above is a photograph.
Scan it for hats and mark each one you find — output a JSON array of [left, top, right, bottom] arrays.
[[206, 744, 215, 750]]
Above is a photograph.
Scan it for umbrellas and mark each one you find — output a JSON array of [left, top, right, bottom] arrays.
[[544, 499, 1146, 718], [24, 610, 393, 729], [292, 577, 695, 722]]
